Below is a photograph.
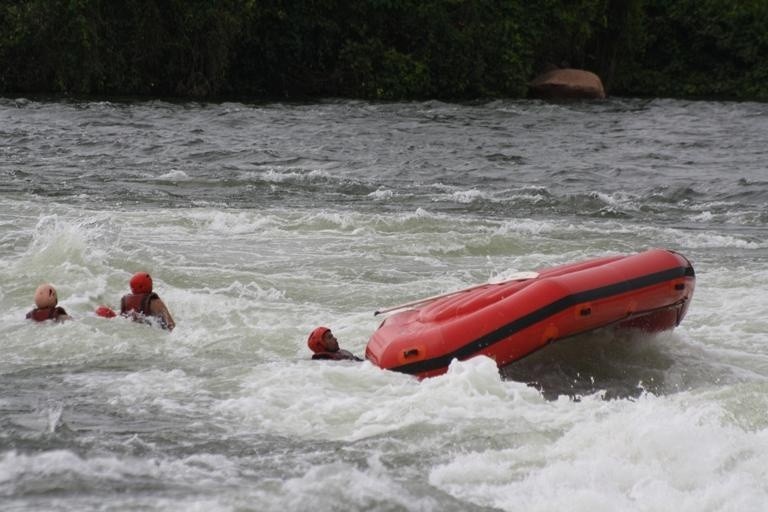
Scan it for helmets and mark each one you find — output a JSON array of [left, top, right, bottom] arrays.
[[34, 284, 57, 309], [307, 327, 331, 353], [130, 272, 152, 294]]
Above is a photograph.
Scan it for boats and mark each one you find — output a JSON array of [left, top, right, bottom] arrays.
[[530, 66, 604, 98], [364, 248, 696, 385]]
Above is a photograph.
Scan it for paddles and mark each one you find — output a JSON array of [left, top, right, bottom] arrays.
[[374, 271, 541, 316]]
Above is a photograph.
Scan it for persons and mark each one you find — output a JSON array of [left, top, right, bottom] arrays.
[[120, 271, 175, 331], [308, 328, 362, 360], [25, 284, 66, 321]]
